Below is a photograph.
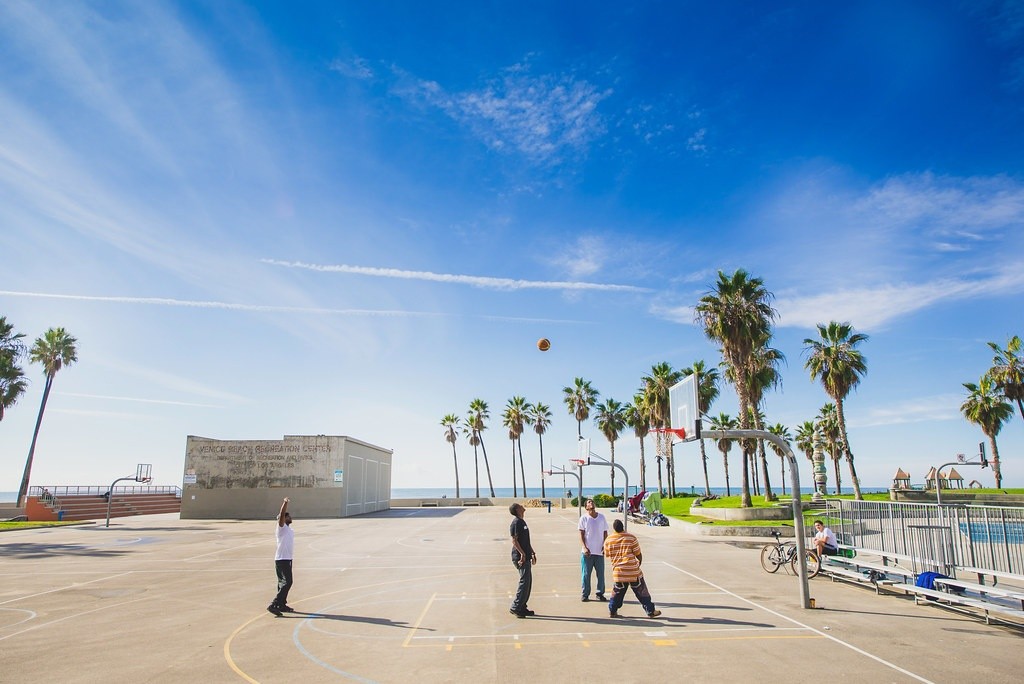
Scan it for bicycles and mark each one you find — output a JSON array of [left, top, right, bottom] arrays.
[[761, 523, 820, 579]]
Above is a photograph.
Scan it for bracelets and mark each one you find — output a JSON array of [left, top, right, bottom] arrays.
[[531, 553, 535, 555]]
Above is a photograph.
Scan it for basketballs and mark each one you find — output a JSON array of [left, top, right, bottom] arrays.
[[537, 338, 551, 351]]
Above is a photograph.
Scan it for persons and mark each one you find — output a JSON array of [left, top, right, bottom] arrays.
[[578, 498, 609, 601], [267, 496, 295, 616], [603, 519, 662, 618], [805, 520, 839, 562], [620, 492, 639, 516], [508, 502, 537, 618], [41, 488, 55, 499]]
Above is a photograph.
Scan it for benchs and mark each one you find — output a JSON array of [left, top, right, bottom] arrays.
[[805, 544, 1024, 627]]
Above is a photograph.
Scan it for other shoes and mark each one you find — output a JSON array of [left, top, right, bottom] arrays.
[[525, 609, 534, 616], [609, 612, 617, 617], [278, 606, 293, 612], [596, 596, 606, 601], [509, 610, 525, 617], [267, 606, 282, 616], [582, 597, 588, 601], [649, 611, 661, 618]]
